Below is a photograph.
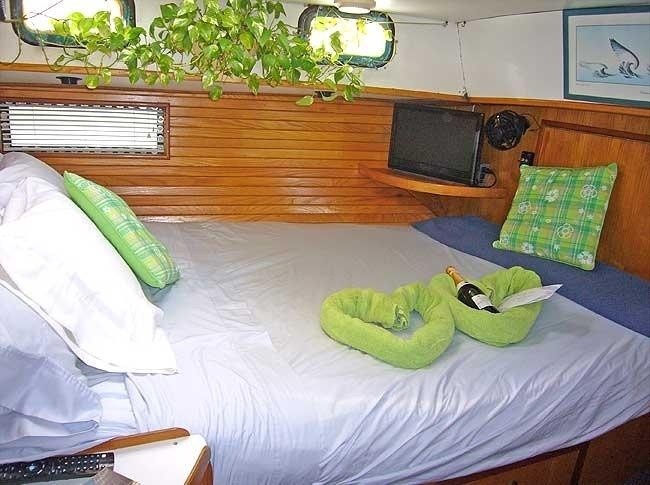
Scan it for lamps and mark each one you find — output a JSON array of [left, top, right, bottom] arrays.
[[334, 0, 376, 14]]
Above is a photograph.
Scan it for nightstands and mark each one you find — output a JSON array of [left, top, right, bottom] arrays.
[[19, 428, 213, 485]]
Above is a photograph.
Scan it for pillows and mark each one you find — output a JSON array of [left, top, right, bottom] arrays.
[[63, 170, 179, 289], [492, 162, 617, 271], [1, 176, 180, 376], [1, 153, 72, 197]]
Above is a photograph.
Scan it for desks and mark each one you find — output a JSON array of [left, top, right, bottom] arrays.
[[359, 163, 508, 217]]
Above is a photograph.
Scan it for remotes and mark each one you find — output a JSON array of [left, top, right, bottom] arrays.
[[0, 452, 114, 485]]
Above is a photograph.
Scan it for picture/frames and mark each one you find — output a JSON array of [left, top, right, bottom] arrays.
[[562, 4, 650, 108]]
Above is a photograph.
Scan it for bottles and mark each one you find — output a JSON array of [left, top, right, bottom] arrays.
[[445, 264, 500, 314]]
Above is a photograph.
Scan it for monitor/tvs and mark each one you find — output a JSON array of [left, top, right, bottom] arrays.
[[388, 102, 485, 187]]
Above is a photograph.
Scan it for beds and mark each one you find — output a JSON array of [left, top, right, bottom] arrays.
[[0, 105, 650, 485]]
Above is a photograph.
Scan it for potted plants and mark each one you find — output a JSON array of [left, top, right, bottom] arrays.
[[0, 0, 398, 105]]
